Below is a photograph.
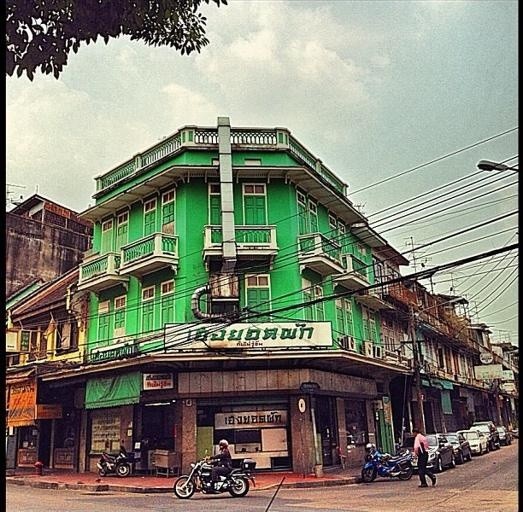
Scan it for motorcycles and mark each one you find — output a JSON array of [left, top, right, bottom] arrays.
[[361, 442, 414, 482], [96, 439, 134, 477], [174, 449, 256, 499]]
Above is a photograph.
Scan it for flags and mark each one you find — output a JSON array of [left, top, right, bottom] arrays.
[[42, 319, 56, 336]]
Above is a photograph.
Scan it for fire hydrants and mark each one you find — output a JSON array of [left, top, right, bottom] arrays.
[[35, 462, 42, 474]]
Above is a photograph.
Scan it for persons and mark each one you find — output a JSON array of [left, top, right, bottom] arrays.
[[206, 439, 233, 485], [412, 428, 436, 487]]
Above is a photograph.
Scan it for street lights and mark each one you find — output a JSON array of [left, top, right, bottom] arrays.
[[411, 299, 468, 436]]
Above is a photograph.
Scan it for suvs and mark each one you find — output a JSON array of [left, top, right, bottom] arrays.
[[470, 421, 500, 450]]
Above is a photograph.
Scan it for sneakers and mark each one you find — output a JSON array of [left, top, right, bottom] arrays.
[[418, 476, 437, 488]]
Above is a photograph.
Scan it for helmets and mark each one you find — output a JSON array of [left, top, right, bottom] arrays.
[[220, 439, 229, 447]]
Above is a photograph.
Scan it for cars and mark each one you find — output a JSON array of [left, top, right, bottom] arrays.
[[495, 426, 511, 445], [396, 430, 489, 473]]
